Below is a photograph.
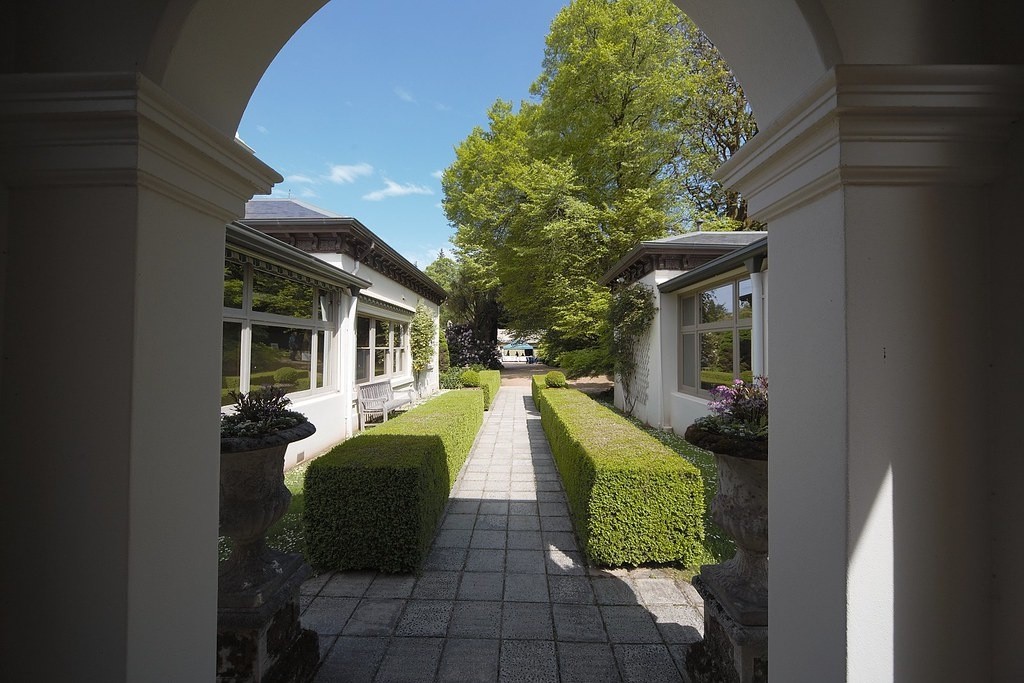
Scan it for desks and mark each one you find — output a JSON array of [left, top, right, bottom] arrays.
[[519, 355, 533, 362], [503, 356, 519, 362]]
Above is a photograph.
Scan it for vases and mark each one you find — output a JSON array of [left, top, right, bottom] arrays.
[[220, 420, 318, 611], [681, 424, 767, 625]]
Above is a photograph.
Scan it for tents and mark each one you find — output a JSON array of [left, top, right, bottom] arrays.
[[502, 339, 533, 361]]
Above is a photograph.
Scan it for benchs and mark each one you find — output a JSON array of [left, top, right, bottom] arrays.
[[357, 378, 416, 431]]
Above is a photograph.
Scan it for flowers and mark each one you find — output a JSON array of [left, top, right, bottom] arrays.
[[694, 367, 769, 435], [222, 384, 305, 436]]
